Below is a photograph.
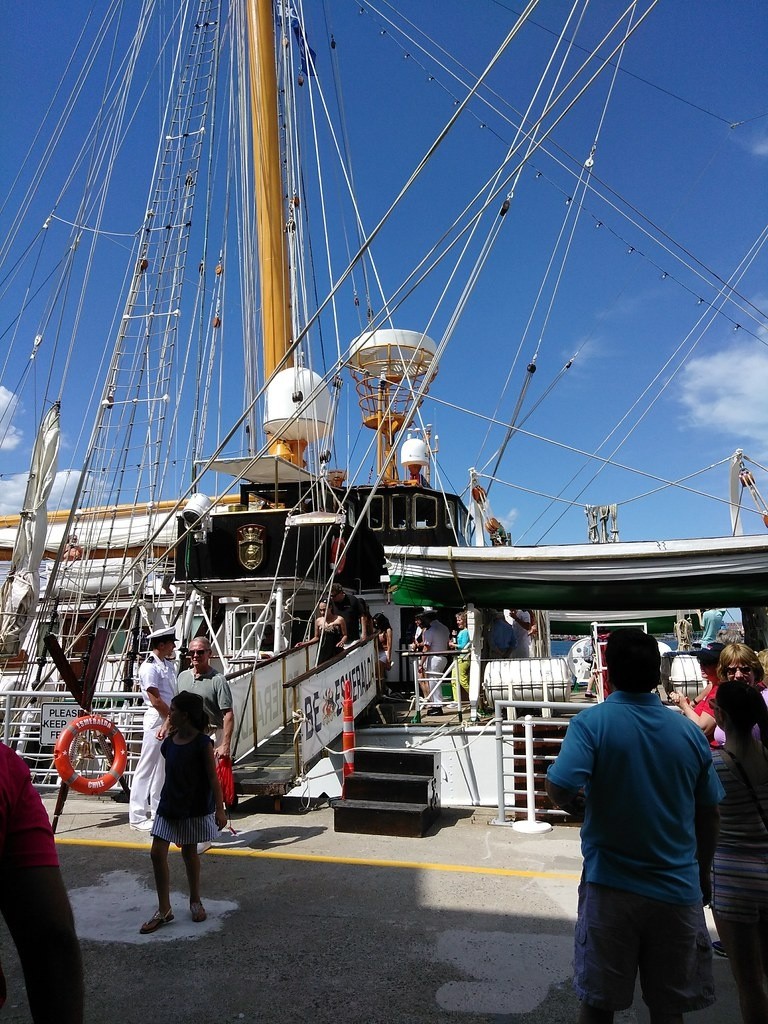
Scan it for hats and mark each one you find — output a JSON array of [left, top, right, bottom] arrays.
[[147, 627, 180, 642], [690, 642, 726, 662]]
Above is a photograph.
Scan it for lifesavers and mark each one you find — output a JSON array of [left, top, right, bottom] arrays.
[[53, 716, 127, 795], [329, 538, 346, 576]]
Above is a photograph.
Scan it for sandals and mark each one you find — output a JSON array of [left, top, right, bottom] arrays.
[[140, 907, 175, 934], [190, 898, 206, 922]]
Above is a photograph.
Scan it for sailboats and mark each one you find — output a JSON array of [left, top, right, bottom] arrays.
[[1, 0, 768, 809]]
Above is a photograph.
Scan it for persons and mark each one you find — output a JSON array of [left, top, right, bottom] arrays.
[[412, 609, 450, 716], [446, 612, 471, 709], [0, 742, 83, 1024], [372, 613, 392, 677], [177, 637, 234, 854], [358, 598, 373, 639], [546, 629, 725, 1024], [711, 682, 768, 1024], [294, 599, 347, 666], [139, 691, 227, 935], [328, 583, 367, 646], [129, 626, 178, 832], [488, 610, 531, 658], [586, 608, 768, 750]]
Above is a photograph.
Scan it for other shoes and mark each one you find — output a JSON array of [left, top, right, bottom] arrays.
[[446, 703, 463, 708], [427, 708, 443, 716], [712, 941, 728, 958], [197, 841, 212, 855], [585, 692, 597, 699], [130, 819, 154, 832]]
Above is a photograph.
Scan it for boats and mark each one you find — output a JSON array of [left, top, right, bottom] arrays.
[[544, 606, 702, 635], [385, 448, 768, 612]]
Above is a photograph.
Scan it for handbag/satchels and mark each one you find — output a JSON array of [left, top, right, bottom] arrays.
[[215, 757, 235, 806]]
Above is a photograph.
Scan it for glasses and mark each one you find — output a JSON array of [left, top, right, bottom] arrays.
[[723, 666, 752, 675], [697, 657, 716, 666], [330, 591, 341, 598], [709, 699, 729, 723], [188, 649, 211, 655]]
